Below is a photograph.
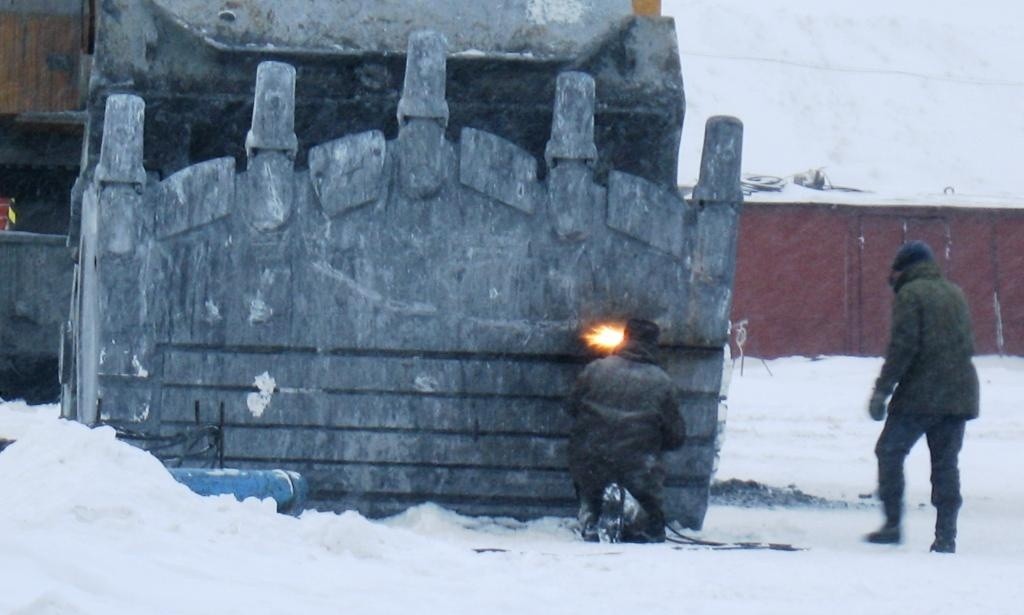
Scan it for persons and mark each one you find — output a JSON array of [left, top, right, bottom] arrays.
[[865, 237, 979, 553], [565, 316, 689, 546]]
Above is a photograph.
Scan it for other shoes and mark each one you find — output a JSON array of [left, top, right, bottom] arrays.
[[596, 482, 646, 544], [582, 512, 599, 544]]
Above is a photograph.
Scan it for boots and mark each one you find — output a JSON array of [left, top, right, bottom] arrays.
[[930, 502, 957, 553], [865, 496, 902, 543]]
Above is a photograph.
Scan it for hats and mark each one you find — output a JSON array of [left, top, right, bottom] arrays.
[[893, 240, 935, 271], [622, 319, 661, 355]]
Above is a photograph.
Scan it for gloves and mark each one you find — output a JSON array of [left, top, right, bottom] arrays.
[[870, 389, 886, 421]]
